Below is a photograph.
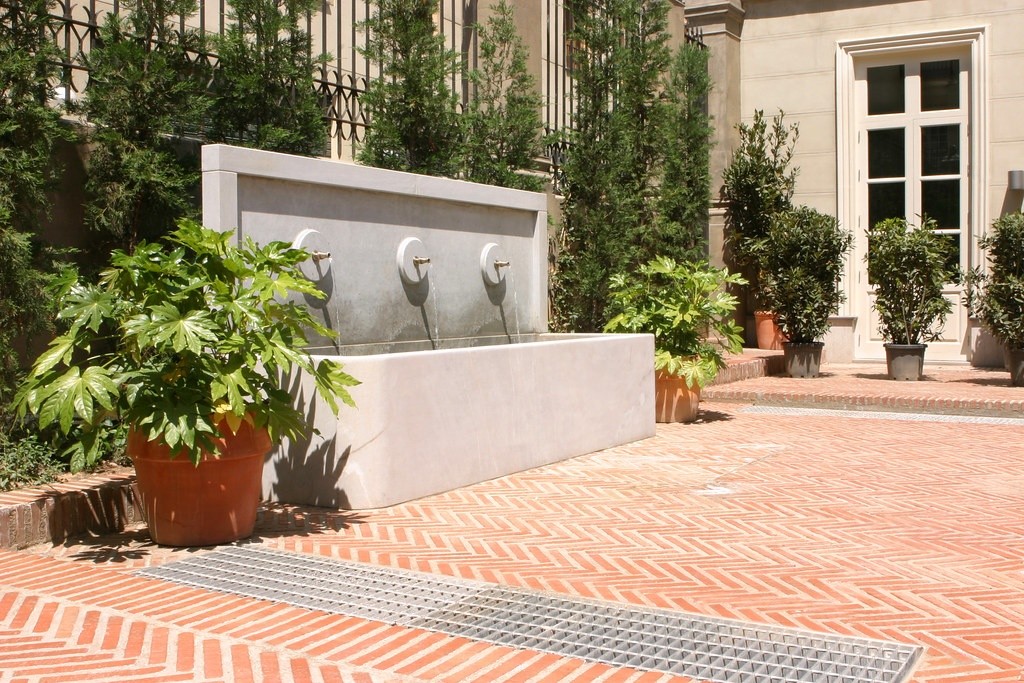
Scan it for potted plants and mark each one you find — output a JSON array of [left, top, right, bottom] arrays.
[[723, 107, 854, 380], [14, 221, 359, 553], [604, 255, 751, 423], [862, 213, 960, 379], [954, 208, 1024, 388]]
